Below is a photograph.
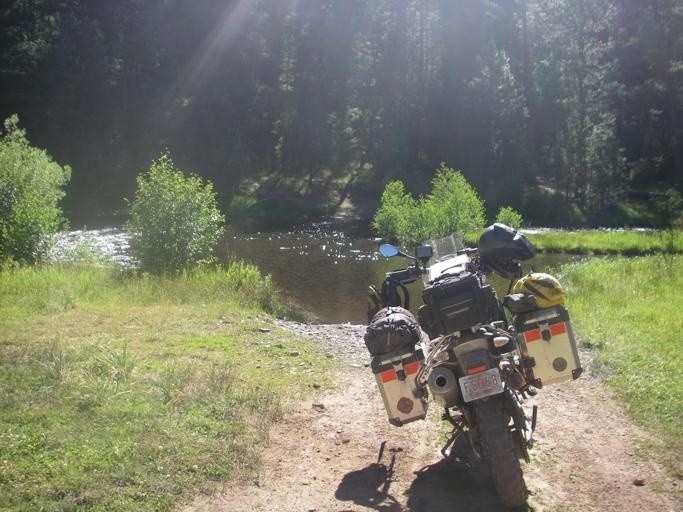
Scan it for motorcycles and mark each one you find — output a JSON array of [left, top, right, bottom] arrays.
[[363, 234, 584, 509]]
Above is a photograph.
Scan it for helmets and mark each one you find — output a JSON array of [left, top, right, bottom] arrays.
[[479, 223, 535, 278]]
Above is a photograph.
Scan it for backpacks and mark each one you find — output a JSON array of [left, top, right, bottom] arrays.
[[368, 277, 409, 324]]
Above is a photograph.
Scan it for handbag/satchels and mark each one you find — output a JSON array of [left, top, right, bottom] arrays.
[[364, 306, 421, 355], [512, 273, 565, 309]]
